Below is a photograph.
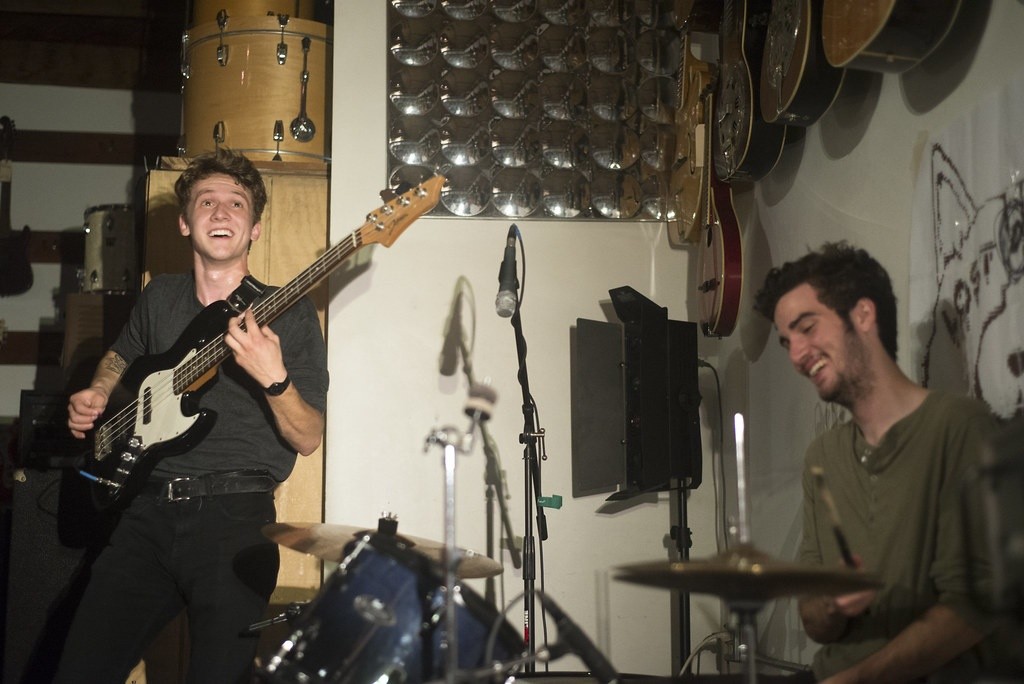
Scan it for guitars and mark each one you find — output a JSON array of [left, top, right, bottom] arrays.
[[666, 0, 957, 338], [86, 174, 448, 512]]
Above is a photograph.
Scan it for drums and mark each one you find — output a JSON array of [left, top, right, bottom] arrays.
[[251, 537, 526, 684]]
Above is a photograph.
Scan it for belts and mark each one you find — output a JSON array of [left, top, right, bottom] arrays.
[[129, 467, 278, 501]]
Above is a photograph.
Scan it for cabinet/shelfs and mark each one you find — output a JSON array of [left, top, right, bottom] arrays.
[[135, 156, 327, 604]]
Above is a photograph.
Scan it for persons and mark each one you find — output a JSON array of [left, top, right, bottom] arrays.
[[54, 151, 328, 684], [757, 240, 1024, 684]]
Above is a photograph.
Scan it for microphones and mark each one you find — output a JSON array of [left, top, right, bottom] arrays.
[[495, 224, 518, 317], [536, 590, 619, 684]]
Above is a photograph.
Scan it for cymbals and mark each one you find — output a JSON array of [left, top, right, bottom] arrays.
[[261, 523, 504, 579], [610, 552, 879, 595]]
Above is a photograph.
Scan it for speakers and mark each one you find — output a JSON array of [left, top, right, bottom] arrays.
[[0, 468, 193, 684]]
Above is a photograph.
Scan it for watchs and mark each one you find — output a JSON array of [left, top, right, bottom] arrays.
[[264, 375, 291, 396]]
